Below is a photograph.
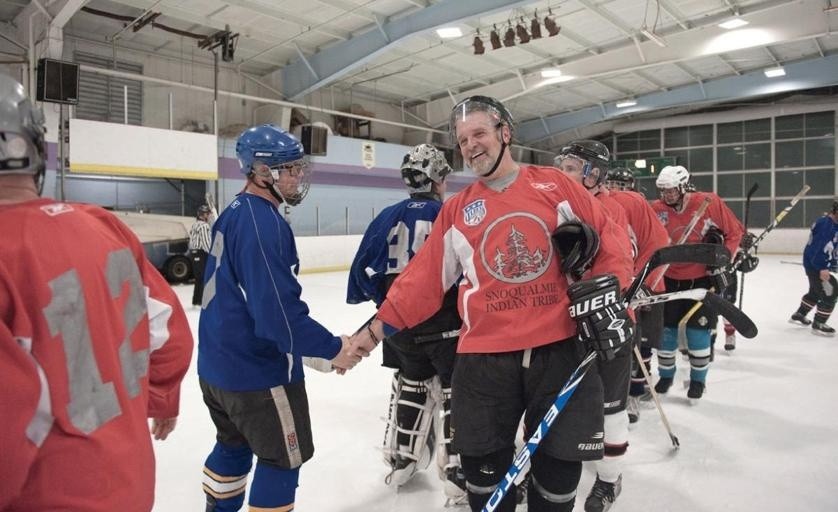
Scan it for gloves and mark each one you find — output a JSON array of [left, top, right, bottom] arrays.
[[733, 250, 759, 273], [566, 273, 636, 362], [707, 266, 734, 295]]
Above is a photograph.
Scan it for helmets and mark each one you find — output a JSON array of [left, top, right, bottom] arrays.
[[656, 165, 690, 195], [553, 138, 611, 187], [449, 95, 514, 145], [401, 143, 454, 194], [197, 205, 212, 221], [0, 72, 47, 195], [604, 167, 637, 191], [235, 123, 311, 208]]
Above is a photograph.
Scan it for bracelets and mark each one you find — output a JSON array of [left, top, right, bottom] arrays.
[[367, 324, 380, 346]]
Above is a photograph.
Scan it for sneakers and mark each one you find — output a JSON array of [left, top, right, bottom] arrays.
[[655, 376, 673, 393], [792, 311, 812, 325], [813, 320, 837, 333], [626, 395, 641, 422], [585, 472, 622, 511], [687, 380, 706, 398], [725, 335, 736, 351]]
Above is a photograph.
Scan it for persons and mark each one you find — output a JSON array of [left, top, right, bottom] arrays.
[[189, 205, 212, 306], [0, 68, 195, 512], [516, 136, 761, 512], [791, 202, 837, 334], [331, 94, 633, 511], [346, 143, 463, 497], [198, 125, 370, 512]]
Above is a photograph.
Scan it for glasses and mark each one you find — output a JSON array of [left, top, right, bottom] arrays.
[[250, 163, 306, 178]]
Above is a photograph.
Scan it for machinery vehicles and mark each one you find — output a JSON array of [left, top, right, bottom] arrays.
[[107, 208, 203, 284]]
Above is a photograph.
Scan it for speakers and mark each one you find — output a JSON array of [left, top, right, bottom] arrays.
[[37, 58, 80, 105], [437, 147, 464, 171], [301, 126, 328, 156]]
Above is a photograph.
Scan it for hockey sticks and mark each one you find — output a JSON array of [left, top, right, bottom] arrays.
[[677, 184, 810, 354], [481, 243, 732, 512], [739, 184, 759, 311], [633, 344, 680, 448], [415, 287, 758, 343]]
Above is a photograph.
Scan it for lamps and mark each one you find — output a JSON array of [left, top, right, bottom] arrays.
[[471, 6, 560, 55], [616, 96, 637, 107], [719, 5, 747, 30], [762, 60, 786, 78]]
